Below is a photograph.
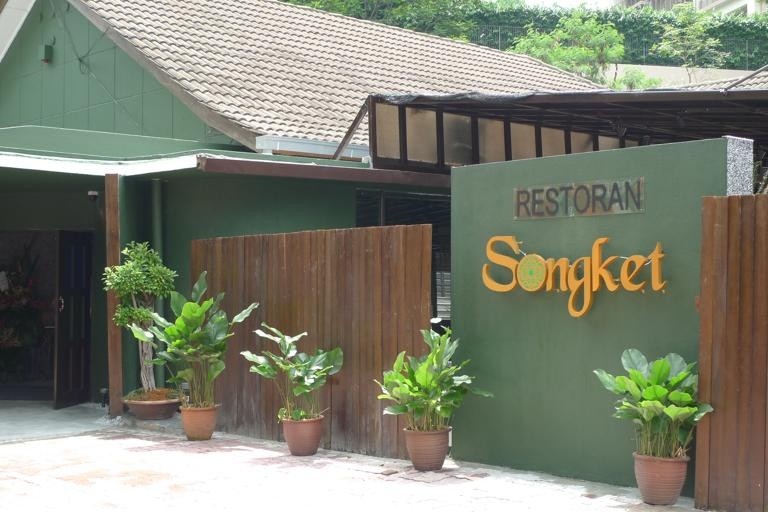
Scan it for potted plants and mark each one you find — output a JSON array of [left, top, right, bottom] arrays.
[[239, 321, 344, 457], [101, 239, 188, 420], [372, 324, 494, 472], [126, 270, 261, 440], [592, 347, 715, 505]]
